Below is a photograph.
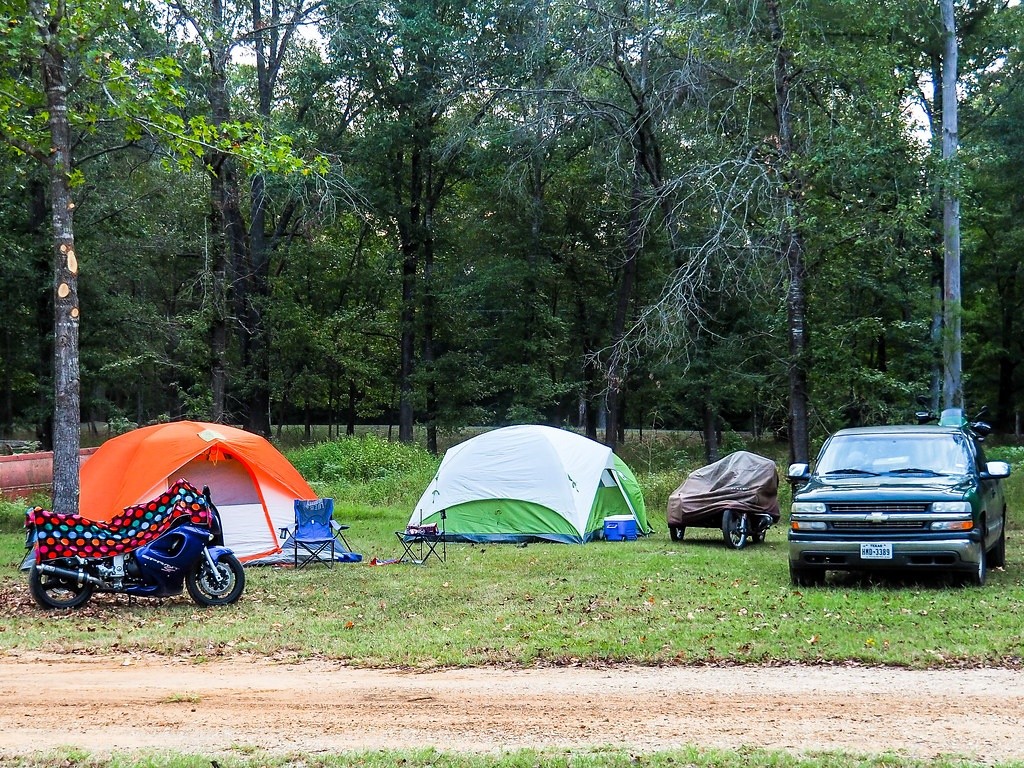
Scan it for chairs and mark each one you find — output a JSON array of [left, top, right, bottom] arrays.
[[278, 498, 350, 570], [842, 445, 875, 472]]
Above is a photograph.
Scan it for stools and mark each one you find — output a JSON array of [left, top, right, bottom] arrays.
[[395, 531, 444, 564]]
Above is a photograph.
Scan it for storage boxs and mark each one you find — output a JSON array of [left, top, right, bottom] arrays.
[[604, 515, 637, 542]]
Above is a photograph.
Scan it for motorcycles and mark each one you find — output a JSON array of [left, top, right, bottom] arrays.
[[915, 405, 994, 436], [15, 477, 246, 612], [666, 501, 774, 549]]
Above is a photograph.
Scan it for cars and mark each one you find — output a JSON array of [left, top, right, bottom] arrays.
[[787, 424, 1012, 585]]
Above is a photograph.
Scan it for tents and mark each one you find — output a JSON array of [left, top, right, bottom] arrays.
[[80, 419, 363, 566], [402, 423, 656, 546]]
